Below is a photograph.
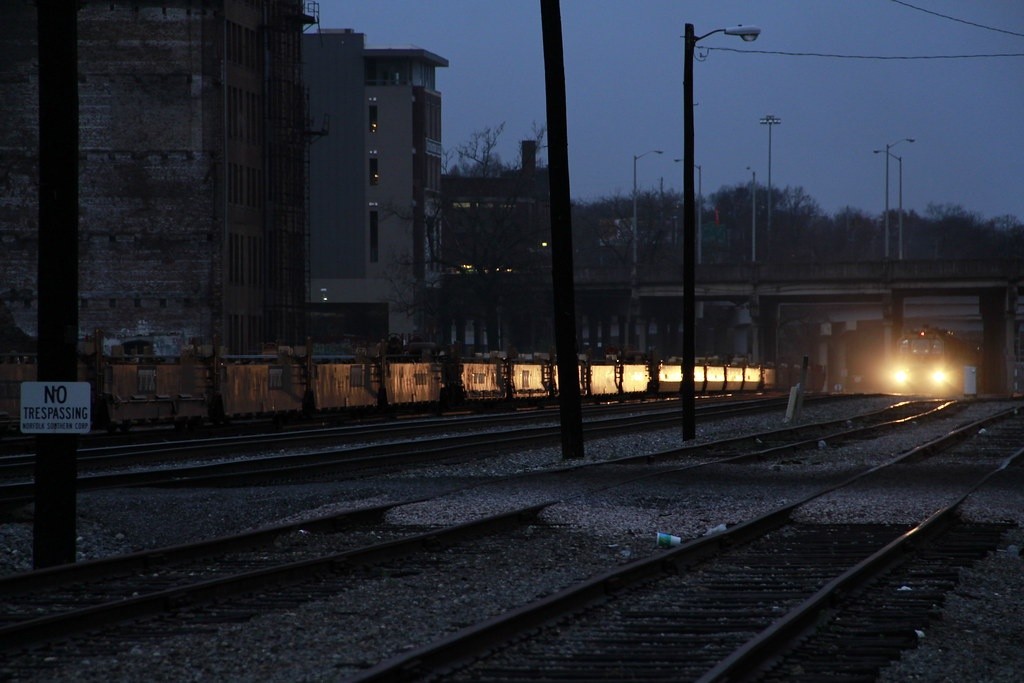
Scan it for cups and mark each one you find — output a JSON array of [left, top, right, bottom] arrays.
[[656, 533, 681, 547]]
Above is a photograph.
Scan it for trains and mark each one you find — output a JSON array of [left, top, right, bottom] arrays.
[[896, 325, 967, 396]]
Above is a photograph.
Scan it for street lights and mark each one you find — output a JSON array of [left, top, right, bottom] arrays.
[[885, 137, 915, 261], [633, 150, 666, 302], [872, 148, 903, 262], [683, 21, 762, 441], [746, 165, 763, 266], [758, 114, 782, 237], [673, 158, 702, 266]]
